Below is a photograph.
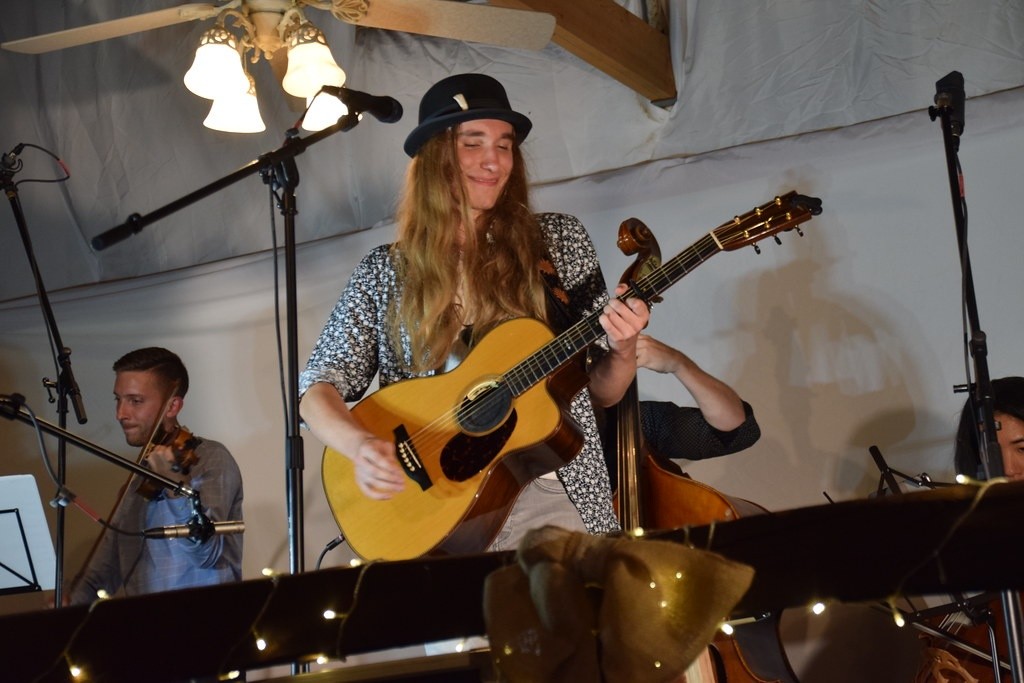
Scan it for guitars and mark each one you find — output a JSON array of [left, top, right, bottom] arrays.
[[321, 190, 824, 562]]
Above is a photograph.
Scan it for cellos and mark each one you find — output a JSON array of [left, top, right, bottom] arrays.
[[586, 217, 796, 680], [899, 588, 1024, 682]]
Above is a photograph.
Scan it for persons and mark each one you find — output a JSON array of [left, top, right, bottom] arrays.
[[301, 74, 654, 654], [951, 378, 1023, 480], [596, 330, 760, 480], [48, 346, 244, 612]]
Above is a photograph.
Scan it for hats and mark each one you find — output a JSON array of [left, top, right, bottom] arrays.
[[404, 74, 532, 156]]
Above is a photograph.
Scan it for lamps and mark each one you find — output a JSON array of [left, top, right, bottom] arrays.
[[182, 6, 363, 133]]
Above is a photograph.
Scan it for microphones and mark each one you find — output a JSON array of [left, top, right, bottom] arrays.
[[935, 71, 965, 137], [323, 85, 403, 123], [141, 521, 246, 539], [0, 143, 25, 190]]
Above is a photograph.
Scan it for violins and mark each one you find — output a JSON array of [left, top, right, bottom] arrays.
[[130, 426, 201, 500]]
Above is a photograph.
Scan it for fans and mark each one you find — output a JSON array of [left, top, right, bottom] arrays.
[[3, 0, 559, 59]]
